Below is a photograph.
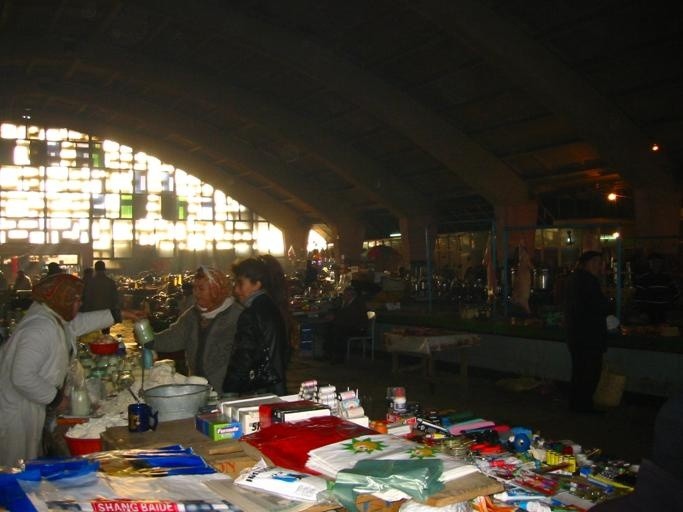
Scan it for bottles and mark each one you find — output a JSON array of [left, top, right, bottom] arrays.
[[5, 307, 23, 337], [71, 330, 127, 416], [393, 387, 407, 413], [546, 445, 588, 473]]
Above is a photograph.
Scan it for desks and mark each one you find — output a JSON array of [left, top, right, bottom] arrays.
[[102, 417, 639, 512]]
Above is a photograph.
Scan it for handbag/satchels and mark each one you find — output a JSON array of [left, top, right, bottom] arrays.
[[243, 311, 278, 393], [42, 406, 72, 458]]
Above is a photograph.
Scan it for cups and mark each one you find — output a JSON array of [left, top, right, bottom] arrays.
[[142, 350, 152, 368], [64, 435, 102, 458], [128, 403, 158, 433]]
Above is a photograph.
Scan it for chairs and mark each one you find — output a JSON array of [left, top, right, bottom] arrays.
[[346, 319, 375, 360]]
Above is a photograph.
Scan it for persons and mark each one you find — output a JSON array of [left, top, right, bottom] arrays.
[[15, 271, 32, 290], [566, 251, 611, 414], [49, 260, 119, 336], [634, 254, 679, 322], [133, 256, 290, 396], [2, 273, 147, 467], [318, 287, 368, 364]]
